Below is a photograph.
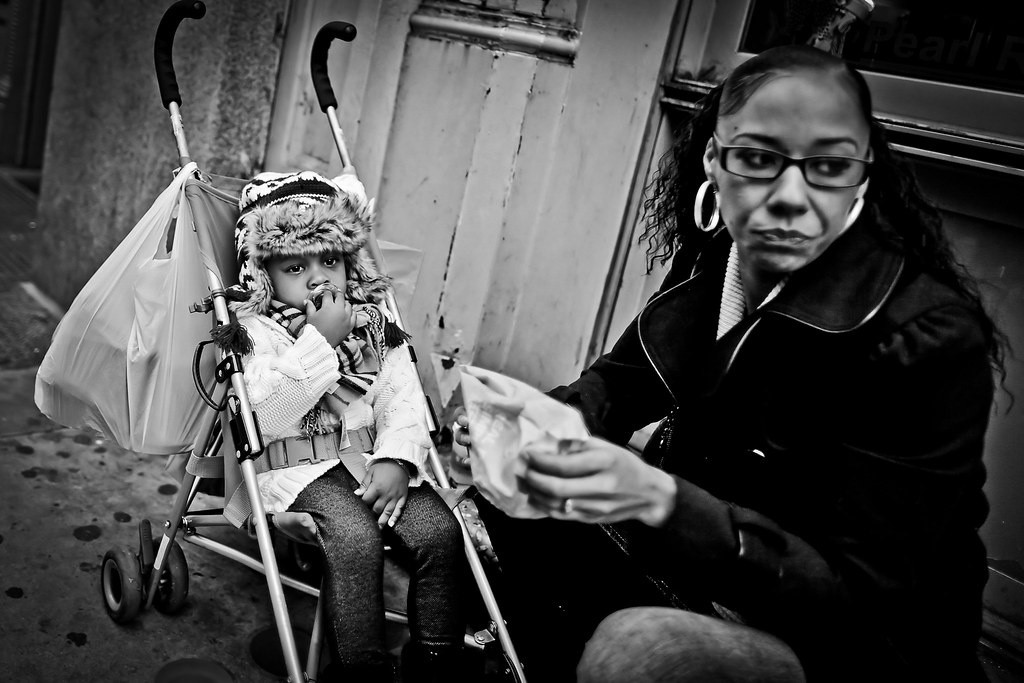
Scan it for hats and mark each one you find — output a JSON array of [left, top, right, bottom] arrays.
[[211, 170, 414, 354]]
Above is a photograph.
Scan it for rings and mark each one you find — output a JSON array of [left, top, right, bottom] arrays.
[[564, 498, 572, 514]]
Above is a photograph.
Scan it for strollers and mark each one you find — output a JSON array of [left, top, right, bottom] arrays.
[[94, 0, 528, 683]]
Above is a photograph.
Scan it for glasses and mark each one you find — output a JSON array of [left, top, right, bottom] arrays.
[[712, 131, 874, 189]]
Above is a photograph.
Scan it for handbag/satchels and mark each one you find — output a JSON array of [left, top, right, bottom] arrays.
[[33, 161, 220, 457]]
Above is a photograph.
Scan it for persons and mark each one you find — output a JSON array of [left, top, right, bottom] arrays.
[[232, 171, 466, 683], [450, 45, 1015, 683]]
